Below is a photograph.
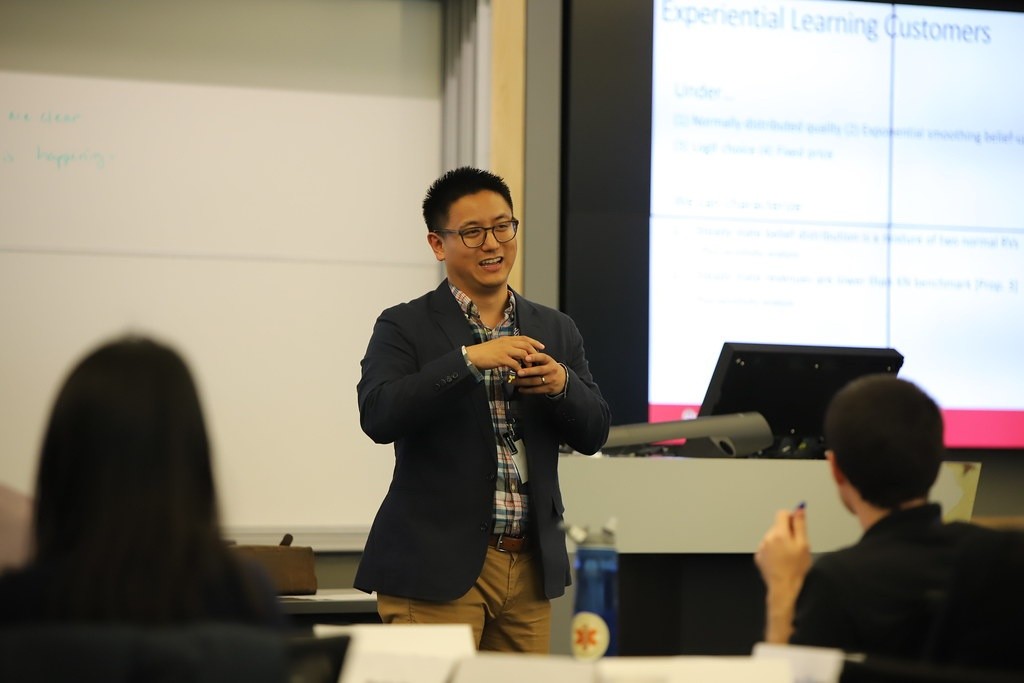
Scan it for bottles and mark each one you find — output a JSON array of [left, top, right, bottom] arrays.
[[572, 528, 622, 658]]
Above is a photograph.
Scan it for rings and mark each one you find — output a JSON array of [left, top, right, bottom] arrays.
[[541, 376, 545, 384]]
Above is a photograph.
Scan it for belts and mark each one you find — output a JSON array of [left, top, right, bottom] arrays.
[[489, 533, 534, 554]]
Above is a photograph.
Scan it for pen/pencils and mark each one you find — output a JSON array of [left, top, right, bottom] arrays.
[[798, 500, 805, 510]]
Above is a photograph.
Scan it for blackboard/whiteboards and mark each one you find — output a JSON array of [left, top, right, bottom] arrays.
[[0, 2, 460, 554]]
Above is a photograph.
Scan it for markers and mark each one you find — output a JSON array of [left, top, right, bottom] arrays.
[[279, 533, 294, 548]]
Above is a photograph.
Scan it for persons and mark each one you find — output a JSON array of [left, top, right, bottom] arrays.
[[756, 375, 1024, 683], [0, 335, 283, 628], [353, 167, 611, 654]]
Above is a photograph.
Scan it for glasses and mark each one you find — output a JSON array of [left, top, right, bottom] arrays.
[[434, 218, 519, 249]]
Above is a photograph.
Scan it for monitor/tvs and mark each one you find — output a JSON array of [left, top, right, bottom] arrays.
[[681, 342, 903, 460]]
